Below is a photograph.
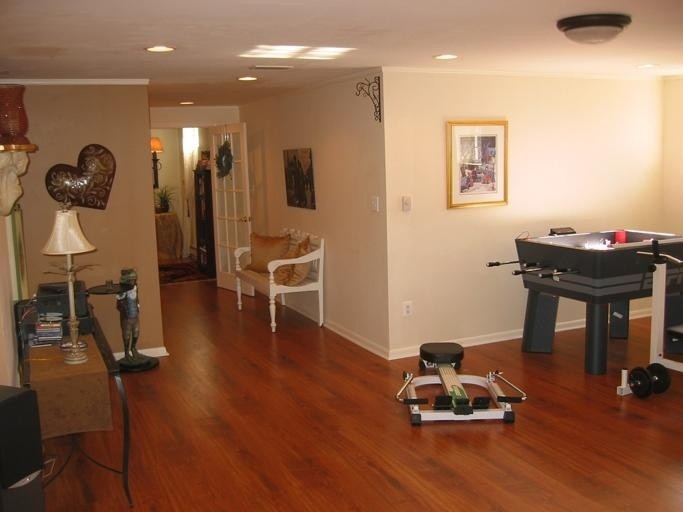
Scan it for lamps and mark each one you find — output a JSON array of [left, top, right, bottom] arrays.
[[41, 209, 99, 367], [149, 136, 164, 187], [557, 11, 631, 45]]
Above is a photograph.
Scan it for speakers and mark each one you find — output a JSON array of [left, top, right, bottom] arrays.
[[0, 470, 44, 511], [0, 384, 44, 488]]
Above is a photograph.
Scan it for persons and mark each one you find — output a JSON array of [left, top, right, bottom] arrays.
[[116, 269, 142, 363]]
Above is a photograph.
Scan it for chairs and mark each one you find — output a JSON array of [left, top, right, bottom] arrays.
[[234, 227, 325, 333]]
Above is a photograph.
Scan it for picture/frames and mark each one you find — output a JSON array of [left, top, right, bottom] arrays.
[[445, 119, 509, 209]]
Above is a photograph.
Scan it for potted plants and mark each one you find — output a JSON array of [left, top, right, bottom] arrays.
[[154, 188, 176, 213]]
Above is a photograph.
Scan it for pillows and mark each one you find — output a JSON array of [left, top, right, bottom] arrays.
[[248, 232, 290, 274], [274, 236, 312, 284]]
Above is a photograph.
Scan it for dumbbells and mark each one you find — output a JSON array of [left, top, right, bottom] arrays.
[[629, 362, 670, 397]]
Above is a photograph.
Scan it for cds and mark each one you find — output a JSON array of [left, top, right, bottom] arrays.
[[35, 312, 62, 340]]
